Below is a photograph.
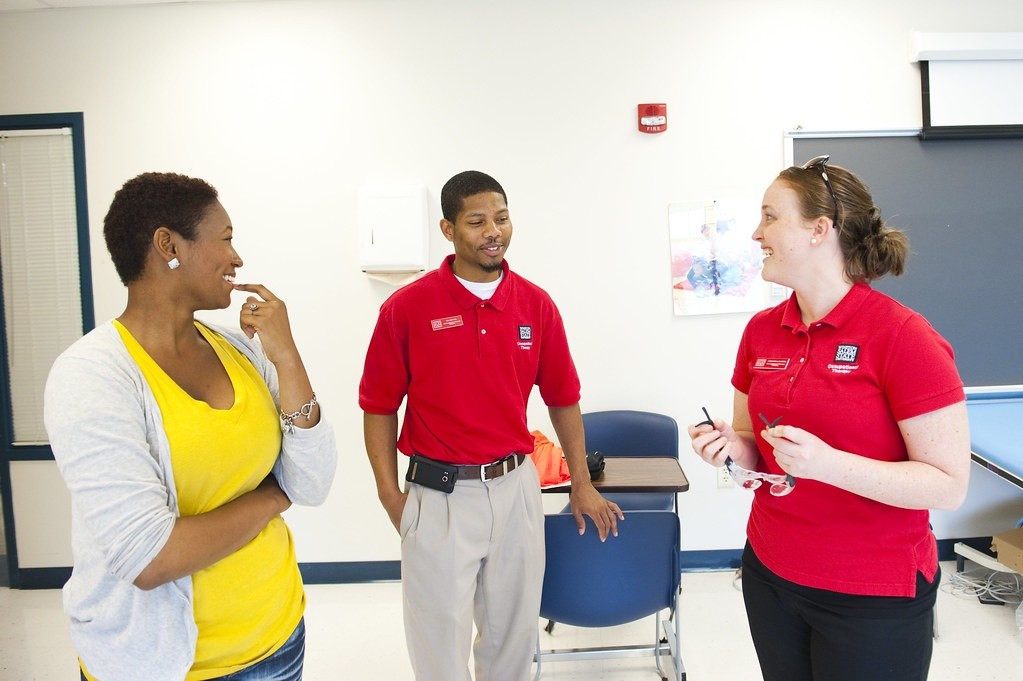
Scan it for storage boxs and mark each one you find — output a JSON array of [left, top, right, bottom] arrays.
[[991, 526, 1023, 574]]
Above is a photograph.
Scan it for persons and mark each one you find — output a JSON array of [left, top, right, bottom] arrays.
[[688, 164, 972, 681], [43, 172, 338, 680], [358, 170, 625, 681]]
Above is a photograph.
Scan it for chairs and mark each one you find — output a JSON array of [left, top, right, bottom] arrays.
[[544, 409, 681, 645], [534, 509, 688, 680]]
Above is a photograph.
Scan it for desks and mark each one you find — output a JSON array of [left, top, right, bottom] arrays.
[[954, 392, 1023, 586], [540, 459, 688, 680]]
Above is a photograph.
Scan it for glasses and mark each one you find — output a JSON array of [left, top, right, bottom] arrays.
[[802, 154, 839, 229], [695, 408, 796, 497]]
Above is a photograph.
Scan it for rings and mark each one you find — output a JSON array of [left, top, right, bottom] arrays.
[[250, 303, 259, 314]]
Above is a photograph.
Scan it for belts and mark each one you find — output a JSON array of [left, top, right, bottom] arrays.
[[456, 454, 526, 483]]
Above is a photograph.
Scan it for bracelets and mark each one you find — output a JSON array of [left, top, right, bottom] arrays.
[[281, 392, 317, 434]]
